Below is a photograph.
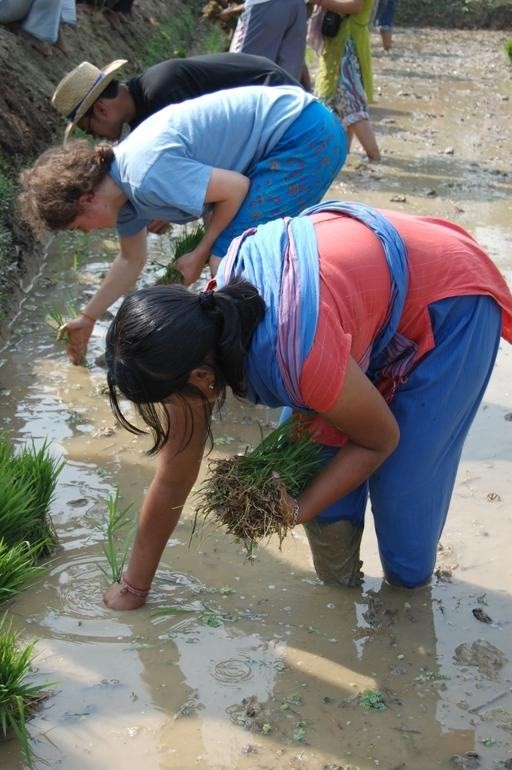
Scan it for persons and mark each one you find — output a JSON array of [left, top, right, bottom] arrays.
[[100, 199, 512, 612], [305, 0, 382, 164], [372, 0, 398, 54], [216, 0, 308, 87], [0, 0, 77, 44], [52, 51, 304, 142], [17, 83, 351, 364]]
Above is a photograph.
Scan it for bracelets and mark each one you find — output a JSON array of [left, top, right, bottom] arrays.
[[80, 310, 97, 323], [119, 574, 150, 598], [287, 497, 300, 532]]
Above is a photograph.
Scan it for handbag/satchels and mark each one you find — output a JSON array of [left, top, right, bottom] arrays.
[[321, 10, 343, 38]]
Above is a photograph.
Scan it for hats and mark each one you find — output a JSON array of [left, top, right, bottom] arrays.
[[50, 58, 128, 140]]
